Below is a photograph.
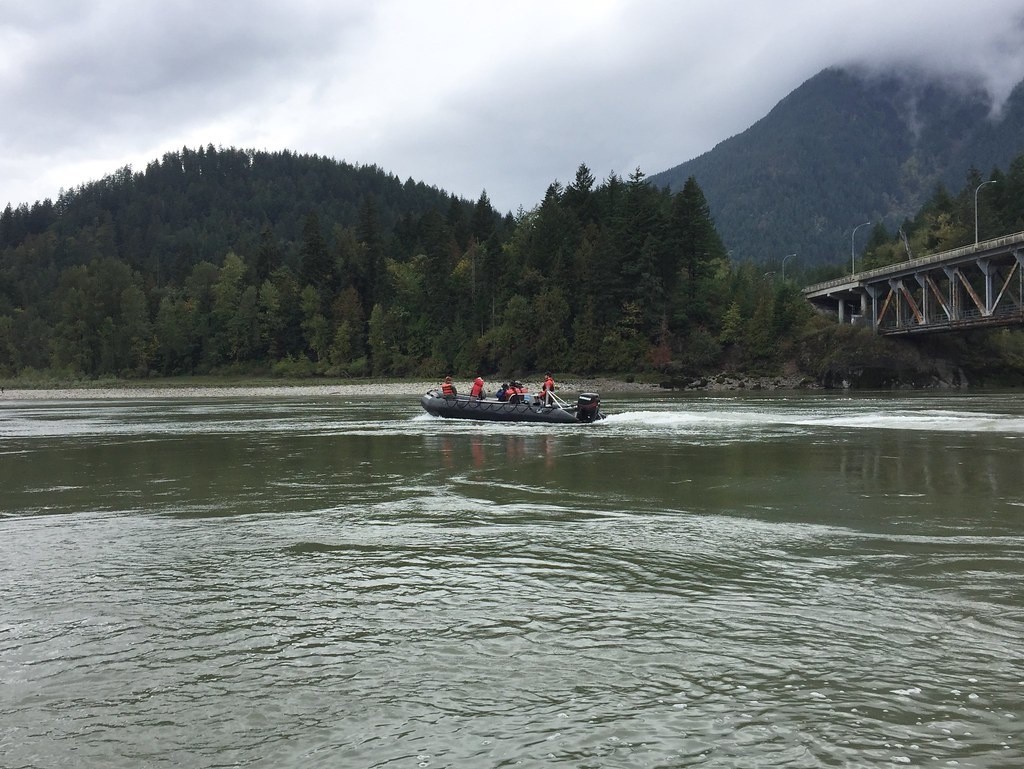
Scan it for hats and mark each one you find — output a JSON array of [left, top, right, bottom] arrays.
[[501, 384, 509, 389]]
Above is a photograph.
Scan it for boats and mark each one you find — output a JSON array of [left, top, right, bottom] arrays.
[[418, 389, 608, 425]]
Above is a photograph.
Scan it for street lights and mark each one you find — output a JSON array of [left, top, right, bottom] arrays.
[[782, 253, 796, 283], [974, 179, 998, 244], [851, 221, 871, 276]]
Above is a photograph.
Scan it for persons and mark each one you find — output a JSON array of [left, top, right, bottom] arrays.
[[469, 377, 486, 400], [442, 376, 457, 400], [496, 380, 528, 401], [537, 371, 555, 406]]
